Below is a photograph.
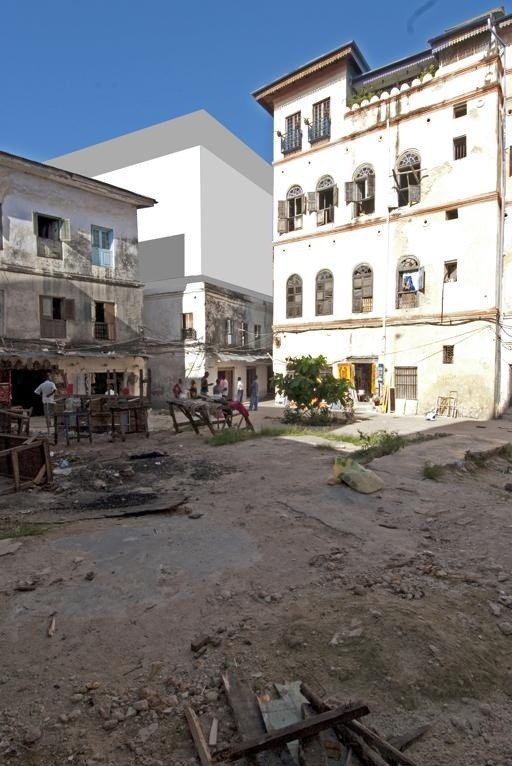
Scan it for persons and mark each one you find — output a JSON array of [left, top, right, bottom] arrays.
[[104, 383, 119, 396], [34, 372, 57, 428], [173, 372, 258, 411]]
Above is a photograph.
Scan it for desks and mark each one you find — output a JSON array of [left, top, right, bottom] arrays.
[[54, 367, 151, 446], [166, 398, 256, 437], [0, 434, 54, 495], [0, 408, 32, 435]]
